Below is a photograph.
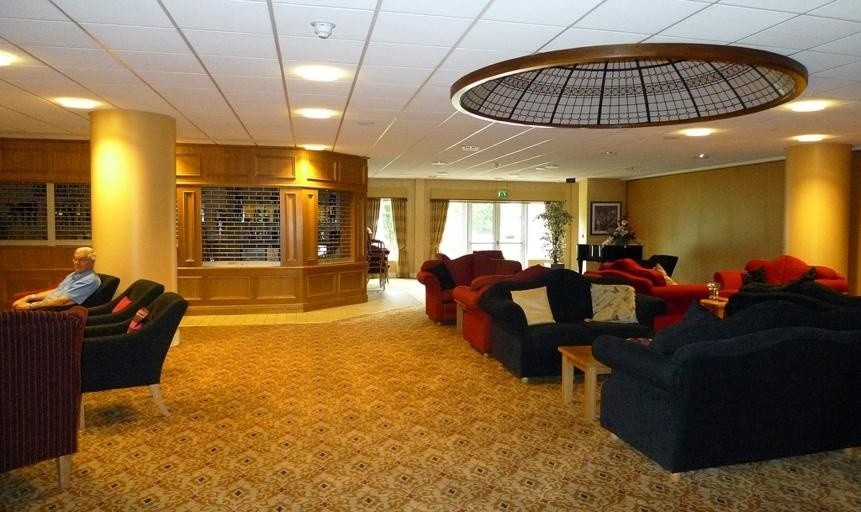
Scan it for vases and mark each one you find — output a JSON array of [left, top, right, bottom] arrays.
[[616, 238, 630, 245]]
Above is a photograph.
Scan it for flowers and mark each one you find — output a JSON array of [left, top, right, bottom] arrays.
[[601, 210, 643, 247]]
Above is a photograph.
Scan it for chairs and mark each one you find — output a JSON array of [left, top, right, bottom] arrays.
[[366, 224, 391, 292], [86, 278, 166, 327], [32, 271, 122, 312], [80, 289, 190, 426], [1, 301, 89, 493]]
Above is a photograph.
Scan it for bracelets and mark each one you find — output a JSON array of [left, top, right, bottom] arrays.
[[32, 294, 35, 300]]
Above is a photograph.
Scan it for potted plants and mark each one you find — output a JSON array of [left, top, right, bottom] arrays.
[[537, 198, 575, 270]]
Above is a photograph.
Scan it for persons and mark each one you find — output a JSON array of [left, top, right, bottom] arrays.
[[12, 247, 102, 310]]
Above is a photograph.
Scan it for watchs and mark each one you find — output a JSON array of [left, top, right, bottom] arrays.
[[29, 304, 33, 310]]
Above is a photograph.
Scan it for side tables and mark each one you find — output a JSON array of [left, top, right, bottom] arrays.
[[454, 299, 466, 336]]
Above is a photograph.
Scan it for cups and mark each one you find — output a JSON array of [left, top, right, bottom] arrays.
[[707, 280, 720, 300]]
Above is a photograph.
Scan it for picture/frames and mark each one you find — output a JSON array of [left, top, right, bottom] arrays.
[[590, 200, 624, 237]]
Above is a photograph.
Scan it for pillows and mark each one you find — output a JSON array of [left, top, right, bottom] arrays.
[[510, 286, 556, 326], [112, 293, 132, 313], [126, 306, 150, 335]]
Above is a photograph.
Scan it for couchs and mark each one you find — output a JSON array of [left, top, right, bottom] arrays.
[[453, 270, 575, 357], [477, 272, 666, 383], [585, 255, 861, 473], [418, 254, 522, 325]]
